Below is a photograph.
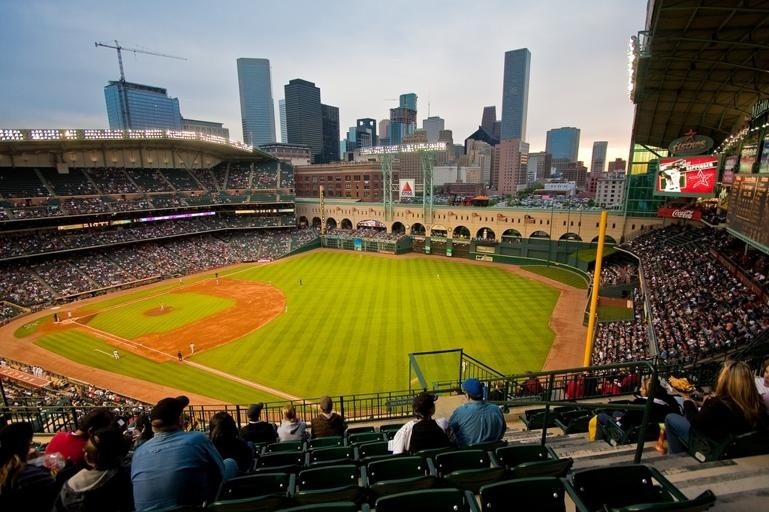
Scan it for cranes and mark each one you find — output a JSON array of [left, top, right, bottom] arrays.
[[93, 38, 189, 128]]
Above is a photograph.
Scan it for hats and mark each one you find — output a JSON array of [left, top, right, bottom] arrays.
[[412, 391, 439, 406], [246, 401, 265, 417], [85, 429, 132, 461], [319, 396, 333, 410], [148, 395, 190, 428], [462, 378, 484, 399]]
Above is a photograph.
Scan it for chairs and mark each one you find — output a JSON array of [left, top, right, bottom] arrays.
[[205, 395, 769, 511]]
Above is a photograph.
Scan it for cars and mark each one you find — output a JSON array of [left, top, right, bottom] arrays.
[[518, 195, 592, 209], [410, 193, 466, 206]]
